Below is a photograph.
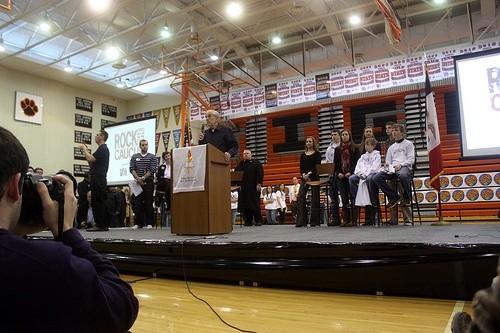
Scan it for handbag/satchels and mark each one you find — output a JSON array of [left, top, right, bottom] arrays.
[[354, 180, 372, 206]]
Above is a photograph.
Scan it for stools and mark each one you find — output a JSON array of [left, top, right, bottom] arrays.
[[304, 156, 422, 227]]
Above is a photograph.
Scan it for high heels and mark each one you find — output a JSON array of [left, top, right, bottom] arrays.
[[295, 222, 308, 227], [310, 222, 316, 226]]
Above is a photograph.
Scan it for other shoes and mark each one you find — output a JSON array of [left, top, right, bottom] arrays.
[[387, 195, 402, 208], [148, 225, 152, 229], [402, 197, 411, 206], [328, 221, 341, 225], [132, 225, 140, 229], [255, 220, 262, 226], [341, 220, 353, 227], [245, 220, 253, 226], [86, 226, 110, 231], [362, 221, 376, 226]]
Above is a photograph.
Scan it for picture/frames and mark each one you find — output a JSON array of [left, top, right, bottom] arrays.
[[14, 89, 44, 125]]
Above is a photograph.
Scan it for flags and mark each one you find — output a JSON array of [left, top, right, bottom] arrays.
[[426, 77, 445, 193]]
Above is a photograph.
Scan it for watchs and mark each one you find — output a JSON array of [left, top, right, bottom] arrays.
[[142, 176, 145, 180]]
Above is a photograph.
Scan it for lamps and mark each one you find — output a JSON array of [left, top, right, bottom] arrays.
[[64, 59, 72, 73], [272, 29, 282, 44], [0, 33, 5, 52], [39, 8, 51, 31], [160, 18, 170, 38], [110, 58, 127, 69], [210, 48, 219, 61]]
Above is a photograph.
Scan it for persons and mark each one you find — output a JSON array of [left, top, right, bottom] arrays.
[[384, 121, 414, 226], [334, 130, 359, 227], [263, 185, 277, 224], [294, 136, 321, 228], [326, 127, 346, 226], [276, 182, 285, 225], [237, 149, 265, 226], [198, 110, 238, 162], [129, 139, 156, 230], [289, 176, 299, 219], [25, 166, 134, 230], [372, 123, 415, 206], [78, 130, 111, 231], [447, 265, 500, 333], [156, 151, 172, 213], [349, 138, 381, 205], [0, 128, 139, 333], [356, 125, 380, 226]]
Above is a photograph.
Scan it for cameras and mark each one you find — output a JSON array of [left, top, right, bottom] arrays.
[[20, 169, 77, 226]]
